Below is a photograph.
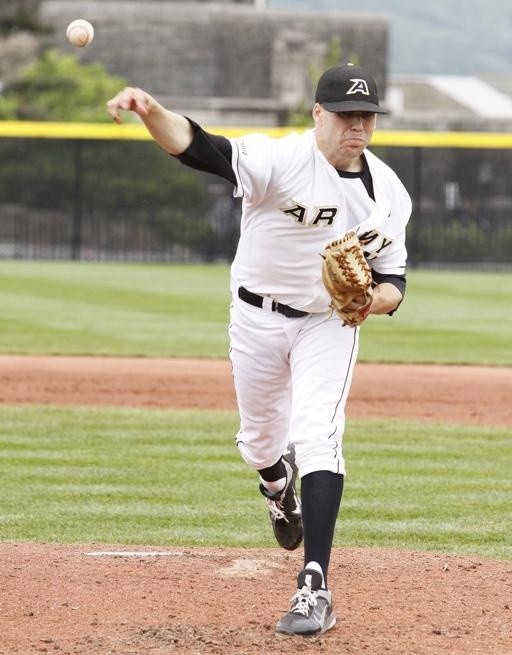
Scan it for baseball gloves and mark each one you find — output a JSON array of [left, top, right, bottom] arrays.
[[320, 233, 373, 328]]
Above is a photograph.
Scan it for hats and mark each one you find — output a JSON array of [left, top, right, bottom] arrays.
[[314, 63, 389, 114]]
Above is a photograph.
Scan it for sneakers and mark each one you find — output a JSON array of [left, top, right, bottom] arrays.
[[259, 441, 303, 551], [274, 569, 336, 642]]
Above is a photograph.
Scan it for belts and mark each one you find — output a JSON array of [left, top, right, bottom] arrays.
[[238, 286, 309, 318]]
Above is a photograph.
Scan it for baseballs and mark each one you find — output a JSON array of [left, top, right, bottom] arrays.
[[64, 18, 96, 50]]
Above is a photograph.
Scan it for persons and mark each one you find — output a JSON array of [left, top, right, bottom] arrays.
[[106, 61, 412, 635]]
